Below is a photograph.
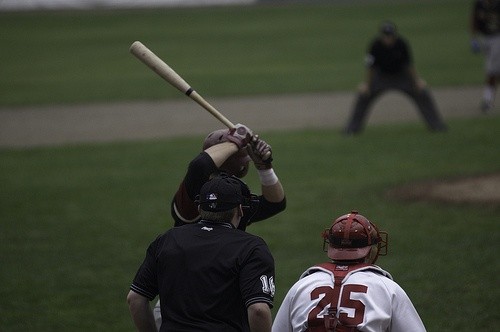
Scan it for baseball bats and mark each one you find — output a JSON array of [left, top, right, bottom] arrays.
[[127, 40, 272, 161]]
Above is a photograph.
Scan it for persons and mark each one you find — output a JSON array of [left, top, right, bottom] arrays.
[[127, 174, 275, 332], [152, 129, 287, 332], [470, 0, 500, 112], [341, 20, 448, 134], [272, 210, 426, 332]]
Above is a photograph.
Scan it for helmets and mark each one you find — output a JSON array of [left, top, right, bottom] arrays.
[[327, 210, 380, 260], [203, 128, 251, 178]]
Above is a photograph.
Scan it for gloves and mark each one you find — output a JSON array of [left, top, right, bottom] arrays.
[[226, 123, 254, 150], [247, 135, 274, 170]]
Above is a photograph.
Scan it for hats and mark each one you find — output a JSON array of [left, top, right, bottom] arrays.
[[197, 176, 244, 212]]
